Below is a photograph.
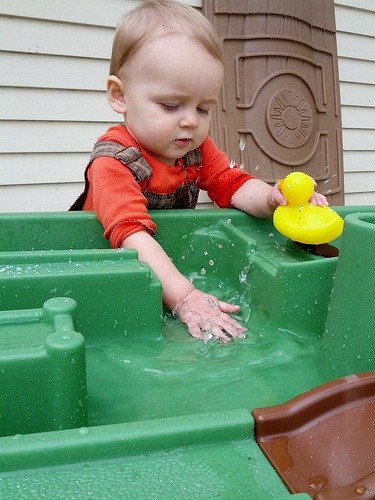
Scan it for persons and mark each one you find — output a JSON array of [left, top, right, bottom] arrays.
[[64, 0, 332, 344]]
